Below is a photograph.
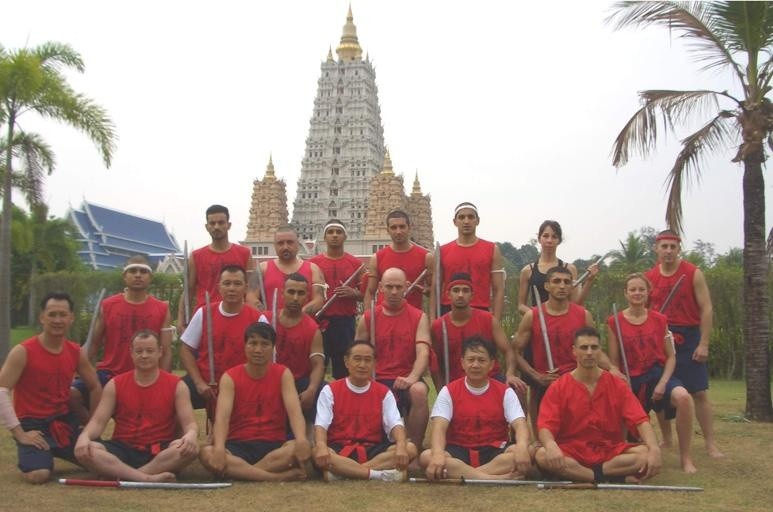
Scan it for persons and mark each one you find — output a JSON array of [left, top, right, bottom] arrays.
[[605, 274, 697, 475], [312, 339, 419, 483], [534, 325, 663, 483], [200, 321, 311, 481], [67, 203, 626, 447], [71, 328, 201, 483], [639, 230, 724, 458], [0, 293, 102, 484], [416, 336, 537, 484]]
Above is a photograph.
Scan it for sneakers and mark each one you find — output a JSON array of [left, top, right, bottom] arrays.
[[379, 469, 407, 483]]
